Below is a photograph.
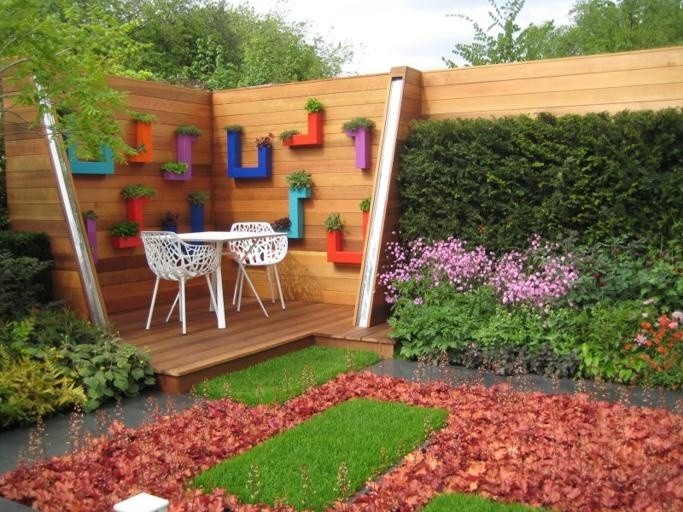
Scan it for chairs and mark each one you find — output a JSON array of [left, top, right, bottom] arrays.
[[226, 219, 291, 314], [136, 229, 220, 337]]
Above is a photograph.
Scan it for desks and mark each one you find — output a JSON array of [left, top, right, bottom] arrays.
[[163, 230, 288, 328]]
